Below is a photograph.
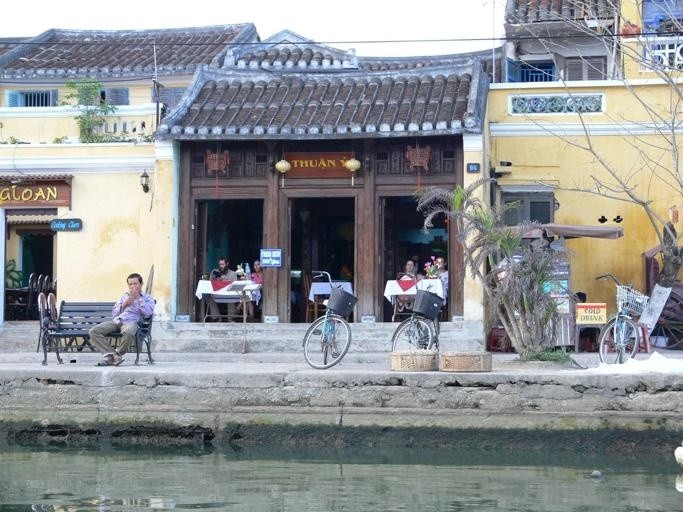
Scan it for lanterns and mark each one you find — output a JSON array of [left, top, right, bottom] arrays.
[[344, 158, 362, 187], [406, 144, 432, 192], [205, 149, 230, 195], [275, 159, 292, 188]]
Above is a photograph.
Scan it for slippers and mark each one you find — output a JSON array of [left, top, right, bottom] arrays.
[[94, 360, 113, 365], [114, 357, 125, 366]]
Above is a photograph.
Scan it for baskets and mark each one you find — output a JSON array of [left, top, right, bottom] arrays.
[[390, 350, 439, 371], [413, 289, 444, 320], [326, 288, 358, 317], [615, 285, 650, 315], [440, 351, 493, 372]]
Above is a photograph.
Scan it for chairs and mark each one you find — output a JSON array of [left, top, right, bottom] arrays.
[[301, 269, 336, 324], [4, 272, 77, 352]]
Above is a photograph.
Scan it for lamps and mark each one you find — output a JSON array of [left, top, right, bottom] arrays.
[[140, 168, 151, 193]]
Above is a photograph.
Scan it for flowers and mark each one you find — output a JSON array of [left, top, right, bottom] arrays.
[[423, 256, 440, 277]]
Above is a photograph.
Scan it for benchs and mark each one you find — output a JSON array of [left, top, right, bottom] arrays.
[[40, 299, 158, 366]]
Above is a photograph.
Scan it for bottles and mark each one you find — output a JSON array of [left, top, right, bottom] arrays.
[[245, 263, 251, 280]]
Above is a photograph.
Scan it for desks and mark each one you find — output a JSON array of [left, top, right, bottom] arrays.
[[384, 278, 444, 323], [310, 281, 354, 324], [197, 279, 258, 323]]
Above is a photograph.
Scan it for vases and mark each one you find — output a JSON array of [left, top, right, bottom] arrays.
[[430, 274, 437, 279]]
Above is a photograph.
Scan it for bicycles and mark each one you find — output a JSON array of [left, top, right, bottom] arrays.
[[299, 269, 357, 370], [390, 270, 445, 353], [594, 272, 651, 364]]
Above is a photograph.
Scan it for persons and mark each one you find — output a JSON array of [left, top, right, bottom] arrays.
[[394, 254, 448, 319], [88, 274, 155, 367], [238, 260, 264, 323], [208, 255, 237, 323], [571, 292, 601, 352]]
